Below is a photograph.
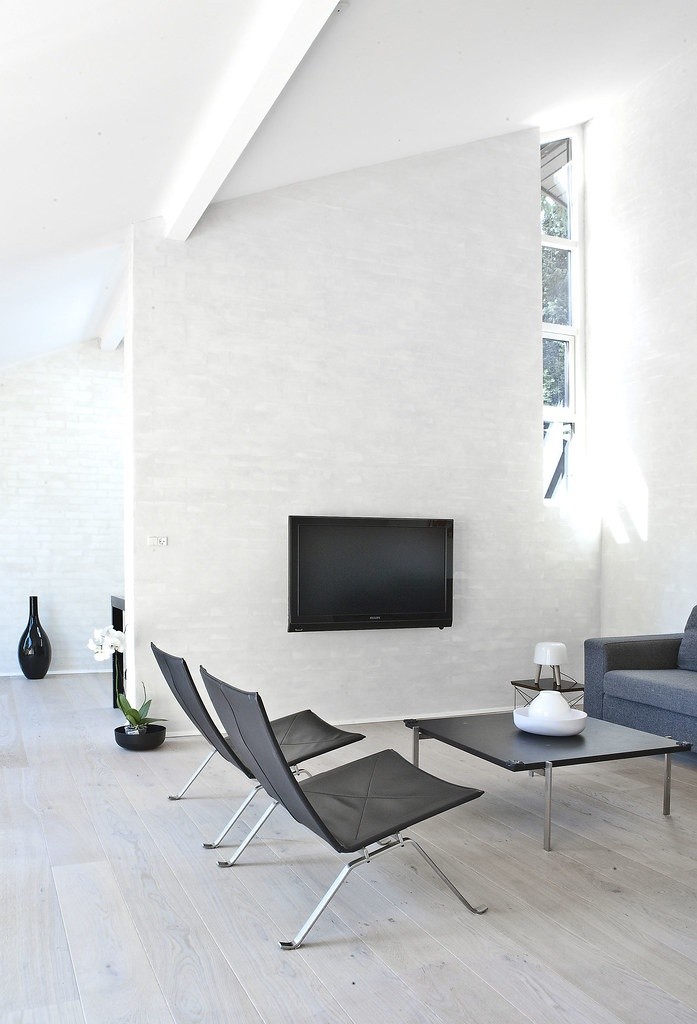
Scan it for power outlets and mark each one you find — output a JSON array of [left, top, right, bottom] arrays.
[[158, 537, 168, 545]]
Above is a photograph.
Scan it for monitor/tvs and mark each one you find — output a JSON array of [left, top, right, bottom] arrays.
[[287, 515, 454, 633]]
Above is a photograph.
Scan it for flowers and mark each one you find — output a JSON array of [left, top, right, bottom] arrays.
[[90, 624, 168, 729]]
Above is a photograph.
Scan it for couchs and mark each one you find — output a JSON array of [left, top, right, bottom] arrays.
[[583, 605, 697, 753]]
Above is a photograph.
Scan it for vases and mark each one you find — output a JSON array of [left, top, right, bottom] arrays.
[[124, 726, 147, 735], [112, 724, 166, 751], [18, 596, 52, 680]]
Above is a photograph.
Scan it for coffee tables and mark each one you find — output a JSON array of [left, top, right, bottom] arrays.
[[403, 713, 694, 852]]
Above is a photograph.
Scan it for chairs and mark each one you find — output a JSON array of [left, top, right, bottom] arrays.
[[149, 640, 365, 847], [196, 665, 491, 949]]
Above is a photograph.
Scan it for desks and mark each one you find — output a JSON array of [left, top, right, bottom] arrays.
[[510, 678, 584, 710]]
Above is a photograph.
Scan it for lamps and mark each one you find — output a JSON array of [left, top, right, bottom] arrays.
[[534, 642, 568, 686]]
